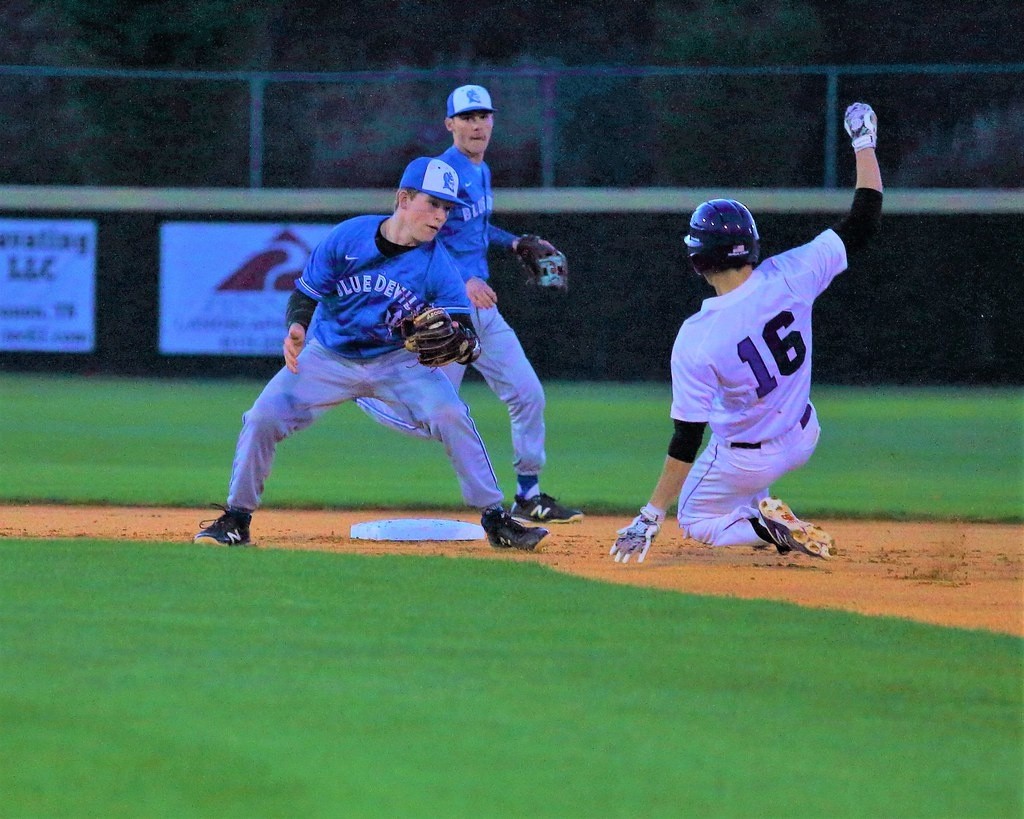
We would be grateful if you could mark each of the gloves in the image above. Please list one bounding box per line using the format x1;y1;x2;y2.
845;103;878;153
610;503;666;564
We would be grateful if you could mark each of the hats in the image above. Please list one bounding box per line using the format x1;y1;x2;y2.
447;84;498;117
399;157;473;210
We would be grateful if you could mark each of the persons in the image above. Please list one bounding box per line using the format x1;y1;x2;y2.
606;102;885;565
193;156;551;552
351;83;587;525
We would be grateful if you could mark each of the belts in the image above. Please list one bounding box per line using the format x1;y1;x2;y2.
732;403;812;449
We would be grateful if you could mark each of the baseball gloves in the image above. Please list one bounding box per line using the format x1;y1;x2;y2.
398;306;478;375
516;232;570;309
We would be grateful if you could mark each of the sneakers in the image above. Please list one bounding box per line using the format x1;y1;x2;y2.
759;496;837;562
510;493;585;523
482;507;551;552
194;502;252;545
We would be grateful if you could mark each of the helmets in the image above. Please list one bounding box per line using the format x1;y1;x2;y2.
684;198;760;275
